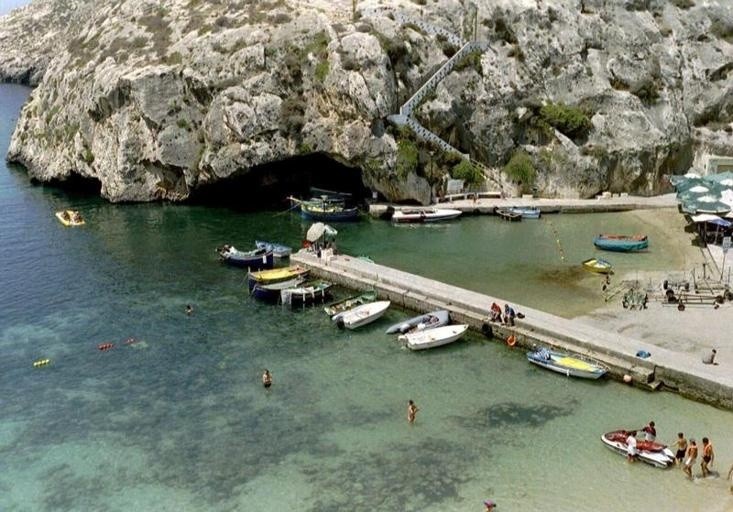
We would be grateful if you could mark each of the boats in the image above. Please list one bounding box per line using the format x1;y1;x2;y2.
300;190;360;221
248;264;333;304
385;309;469;350
323;288;391;330
582;258;614;275
55;209;85;227
593;233;648;253
392;206;462;223
526;348;608;379
601;430;675;468
495;207;540;222
215;240;293;267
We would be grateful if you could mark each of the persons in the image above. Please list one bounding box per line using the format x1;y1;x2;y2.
681;439;698;478
263;369;271;388
185;305;193;313
503;304;516;325
491;302;502;322
626;431;638;462
407;400;418;423
640;421;657;443
700;437;715;477
670;432;688;464
727;465;733;493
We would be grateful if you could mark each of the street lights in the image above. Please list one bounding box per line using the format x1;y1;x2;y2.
427;150;435;205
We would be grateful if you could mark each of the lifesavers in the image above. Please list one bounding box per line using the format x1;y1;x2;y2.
507;336;516;346
